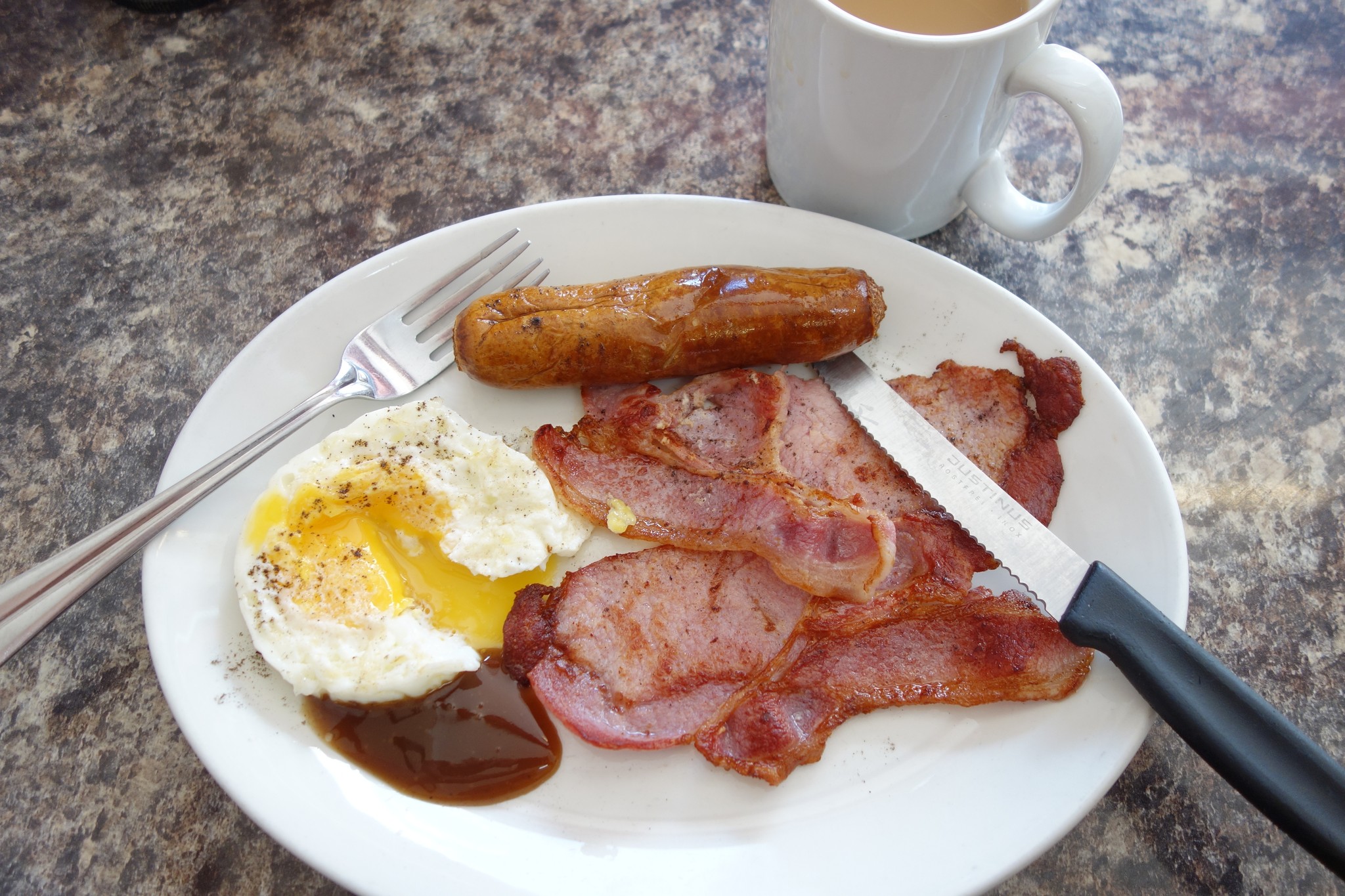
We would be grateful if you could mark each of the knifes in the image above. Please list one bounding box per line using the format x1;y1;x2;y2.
804;353;1345;882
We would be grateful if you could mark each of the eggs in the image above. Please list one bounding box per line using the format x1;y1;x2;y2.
232;395;593;704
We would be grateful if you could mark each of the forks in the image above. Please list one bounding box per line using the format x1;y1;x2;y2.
1;222;554;667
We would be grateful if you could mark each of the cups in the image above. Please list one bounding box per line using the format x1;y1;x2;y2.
764;0;1124;241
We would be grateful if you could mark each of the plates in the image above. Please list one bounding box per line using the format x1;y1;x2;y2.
140;192;1193;896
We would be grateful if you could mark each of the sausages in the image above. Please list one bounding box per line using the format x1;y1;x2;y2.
453;267;888;388
